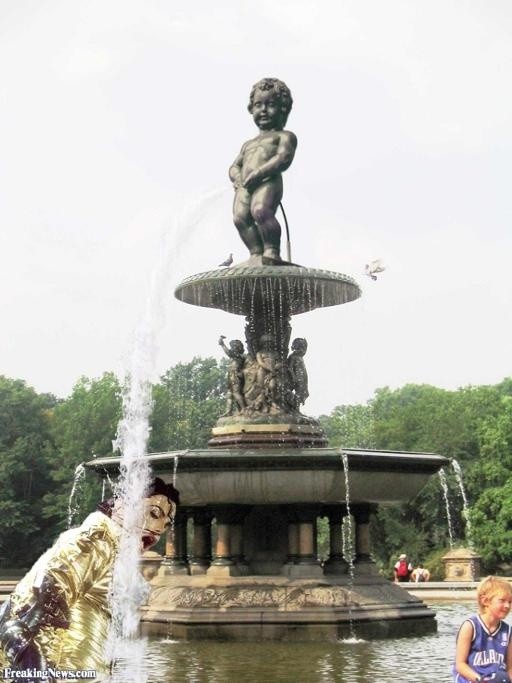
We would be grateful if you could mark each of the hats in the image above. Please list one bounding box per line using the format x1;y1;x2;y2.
399;554;407;559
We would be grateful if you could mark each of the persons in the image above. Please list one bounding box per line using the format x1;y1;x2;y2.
452;575;512;683
230;79;298;265
256;333;284;413
287;338;309;410
0;478;182;683
393;553;413;582
217;336;249;416
410;564;431;583
